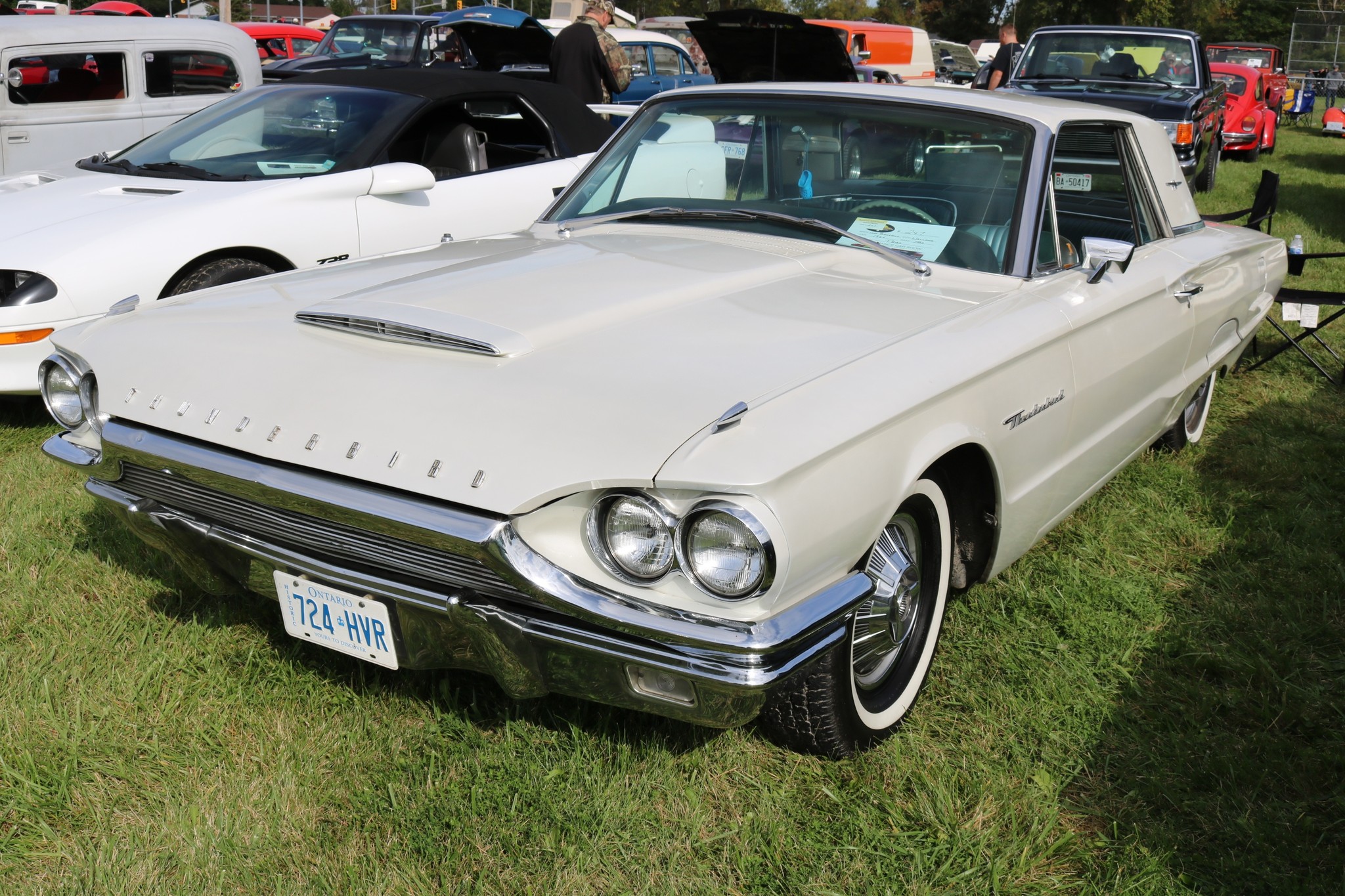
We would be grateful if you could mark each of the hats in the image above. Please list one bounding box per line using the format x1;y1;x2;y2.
587;0;618;27
1325;66;1328;69
1182;52;1191;60
278;15;281;18
281;18;286;22
1309;68;1313;71
272;19;278;23
293;18;298;22
1168;53;1176;62
1320;66;1324;69
1165;50;1173;58
1333;65;1341;69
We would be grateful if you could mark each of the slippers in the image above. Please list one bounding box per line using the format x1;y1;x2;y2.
798;170;813;200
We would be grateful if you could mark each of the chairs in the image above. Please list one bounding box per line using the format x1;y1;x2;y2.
956;224;1079;272
1199;170;1345;385
780;193;957;226
1005;209;1150;245
421;120;488;177
51;66;125;102
333;121;389;166
1282;89;1315;128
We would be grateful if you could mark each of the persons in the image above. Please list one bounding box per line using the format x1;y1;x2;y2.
549;0;632;121
1155;50;1191;78
985;23;1023;91
1305;65;1343;110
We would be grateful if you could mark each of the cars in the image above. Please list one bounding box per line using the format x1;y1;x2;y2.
1;3;1294;393
36;82;1288;762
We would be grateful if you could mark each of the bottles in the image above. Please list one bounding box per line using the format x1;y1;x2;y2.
1291;236;1304;253
